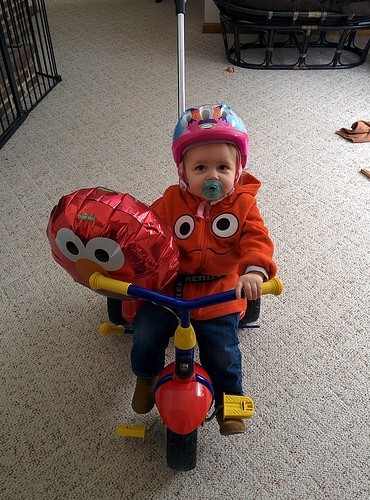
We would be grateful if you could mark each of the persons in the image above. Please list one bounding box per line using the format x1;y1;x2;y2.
121;103;278;436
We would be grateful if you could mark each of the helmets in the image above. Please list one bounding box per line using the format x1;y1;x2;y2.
170;101;251;170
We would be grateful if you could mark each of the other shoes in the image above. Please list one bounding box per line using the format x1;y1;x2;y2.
131;376;154;414
215;417;247;435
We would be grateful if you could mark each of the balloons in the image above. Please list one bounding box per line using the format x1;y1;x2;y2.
45;186;181;297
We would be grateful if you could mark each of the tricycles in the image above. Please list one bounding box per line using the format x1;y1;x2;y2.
89;0;283;473
